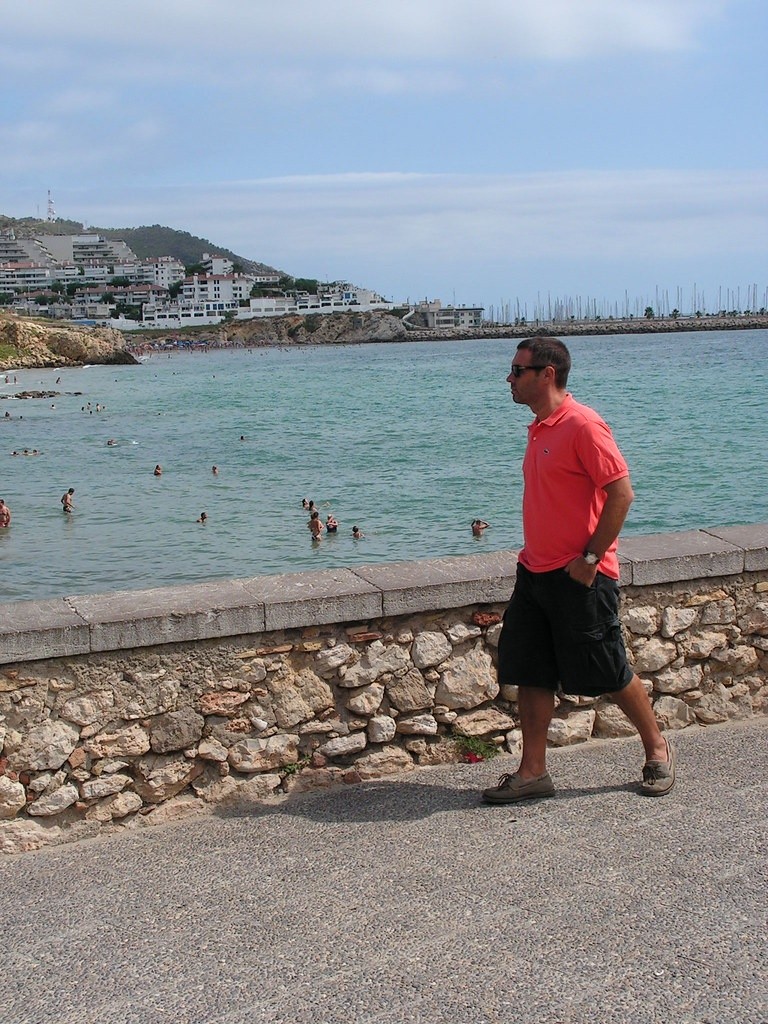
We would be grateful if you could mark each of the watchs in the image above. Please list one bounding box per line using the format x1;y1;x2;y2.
583;551;600;565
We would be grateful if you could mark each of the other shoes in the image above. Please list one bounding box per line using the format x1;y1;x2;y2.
641;738;675;796
482;772;555;803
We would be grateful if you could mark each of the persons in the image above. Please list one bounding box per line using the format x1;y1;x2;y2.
307;511;324;542
470;518;490;536
479;336;677;806
301;498;309;509
351;526;364;539
212;466;218;476
153;464;162;476
196;512;209;523
306;500;316;512
0;500;11;528
325;514;338;533
60;488;75;513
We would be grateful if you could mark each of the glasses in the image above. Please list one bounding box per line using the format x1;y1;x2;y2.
512;365;545;376
